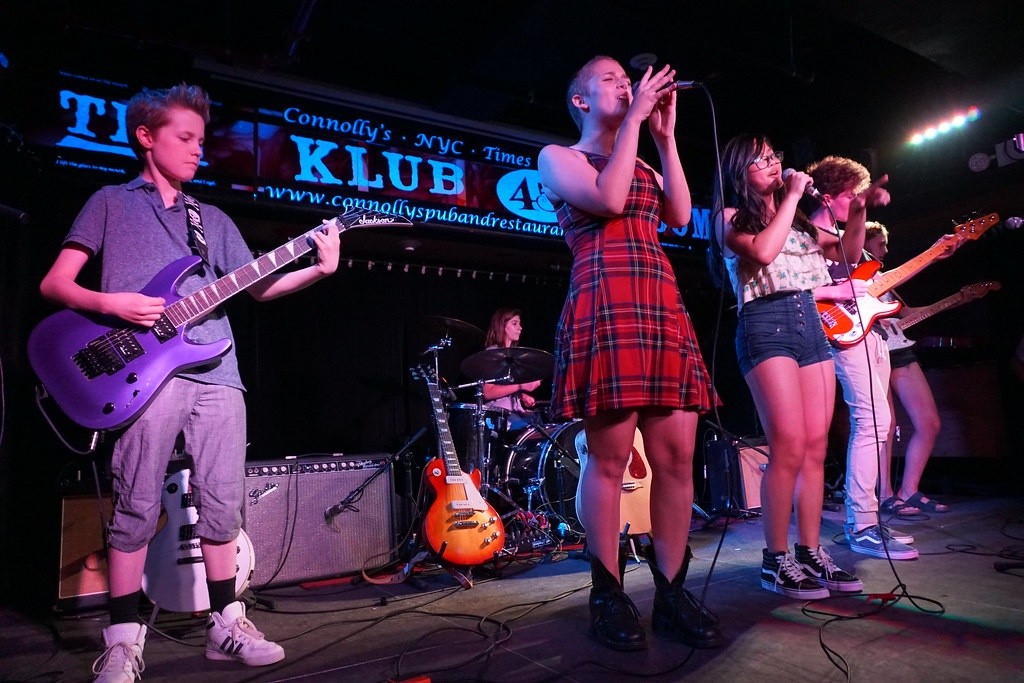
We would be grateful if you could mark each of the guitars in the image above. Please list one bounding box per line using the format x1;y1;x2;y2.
575;425;652;535
409;362;505;567
815;208;1000;350
26;207;414;435
879;280;1001;351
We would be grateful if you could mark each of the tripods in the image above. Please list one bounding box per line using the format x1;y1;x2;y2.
340;424;428;586
449;361;587;563
702;419;773;529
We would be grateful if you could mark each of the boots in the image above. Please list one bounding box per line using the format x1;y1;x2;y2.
644;541;720;646
583;541;645;650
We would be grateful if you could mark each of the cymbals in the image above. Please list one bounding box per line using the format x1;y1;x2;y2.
415;315;486;342
461;347;555;382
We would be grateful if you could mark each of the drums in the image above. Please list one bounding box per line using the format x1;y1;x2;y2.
506;422;586;538
446;402;510;490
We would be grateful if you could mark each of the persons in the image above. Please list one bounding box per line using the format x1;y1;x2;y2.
709;132;889;600
802;157;969;560
538;56;724;647
863;223;990;513
482;308;540;410
39;85;340;683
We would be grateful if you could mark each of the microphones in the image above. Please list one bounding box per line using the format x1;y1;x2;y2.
782;168;827;202
632;80;703;94
441;378;457;403
325;503;349;518
758;463;768;472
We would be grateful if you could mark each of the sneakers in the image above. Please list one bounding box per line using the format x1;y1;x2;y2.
90;623;148;683
763;547;834;600
205;601;285;666
849;526;919;561
843;517;914;546
796;541;863;591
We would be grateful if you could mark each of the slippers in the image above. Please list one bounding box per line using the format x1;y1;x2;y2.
900;492;947;513
879;494;923;516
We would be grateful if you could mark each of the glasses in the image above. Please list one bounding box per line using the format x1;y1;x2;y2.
749;152;784;169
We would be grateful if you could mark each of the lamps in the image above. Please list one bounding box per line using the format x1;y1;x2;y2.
969;134;1024;171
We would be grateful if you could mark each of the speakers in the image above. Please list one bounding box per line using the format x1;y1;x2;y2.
706;434;770;518
240;451;399;590
53;480;115;612
